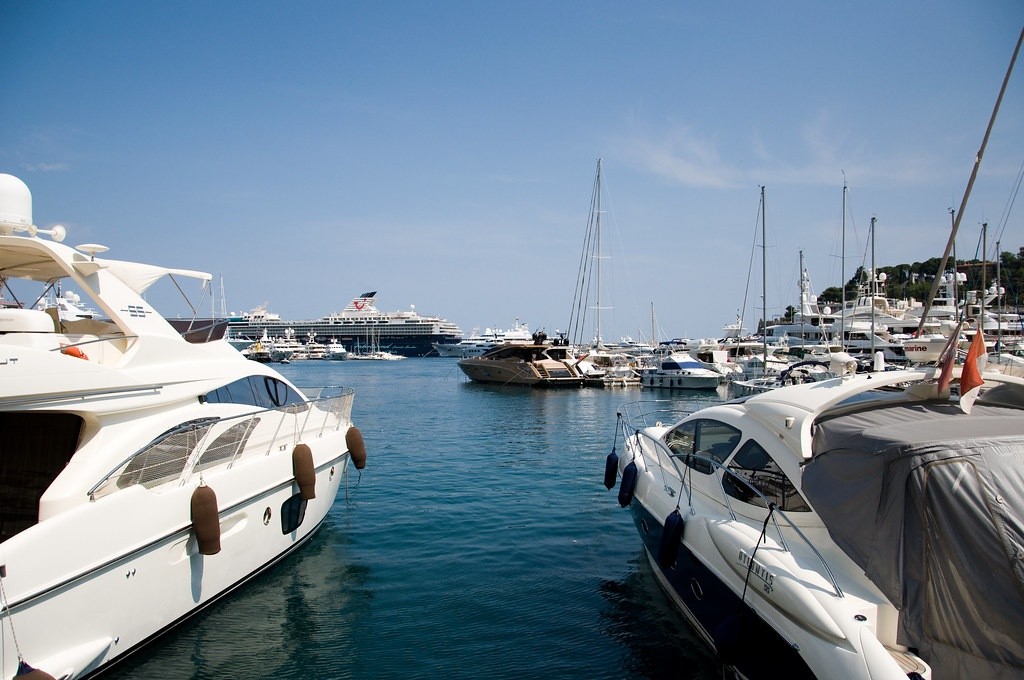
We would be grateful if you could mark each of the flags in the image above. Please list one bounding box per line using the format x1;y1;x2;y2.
960;326;988;415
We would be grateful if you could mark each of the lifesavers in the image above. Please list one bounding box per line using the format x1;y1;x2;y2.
63;346;88;360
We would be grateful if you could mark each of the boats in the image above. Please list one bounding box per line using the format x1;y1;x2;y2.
604;353;1024;680
0;175;367;680
240;325;408;363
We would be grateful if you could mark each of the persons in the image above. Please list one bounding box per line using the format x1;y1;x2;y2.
534;332;543;345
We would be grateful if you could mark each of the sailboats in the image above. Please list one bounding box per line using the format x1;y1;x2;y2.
454;26;1024;393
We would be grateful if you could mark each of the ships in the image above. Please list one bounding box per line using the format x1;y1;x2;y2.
223;292;461;356
